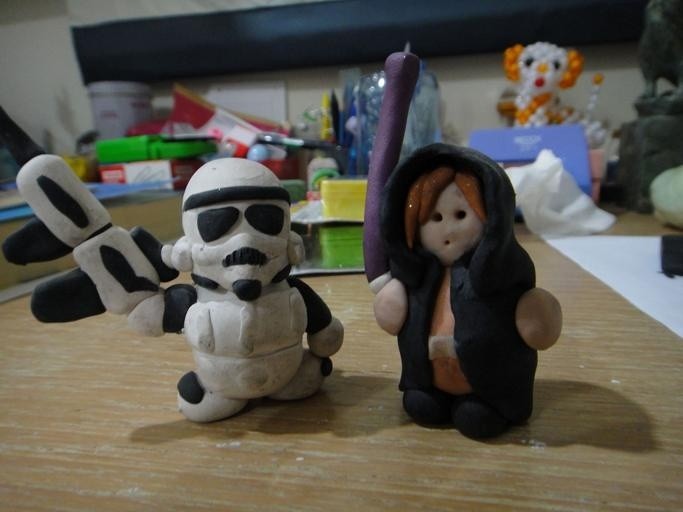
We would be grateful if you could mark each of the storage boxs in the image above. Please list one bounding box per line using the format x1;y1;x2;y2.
320;179;367;219
98;157;202;191
317;225;366;269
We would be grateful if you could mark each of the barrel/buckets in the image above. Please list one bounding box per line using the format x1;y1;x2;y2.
86;80;152;137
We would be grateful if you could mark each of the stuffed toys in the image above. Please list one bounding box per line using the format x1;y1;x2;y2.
2;149;343;424
362;45;562;441
